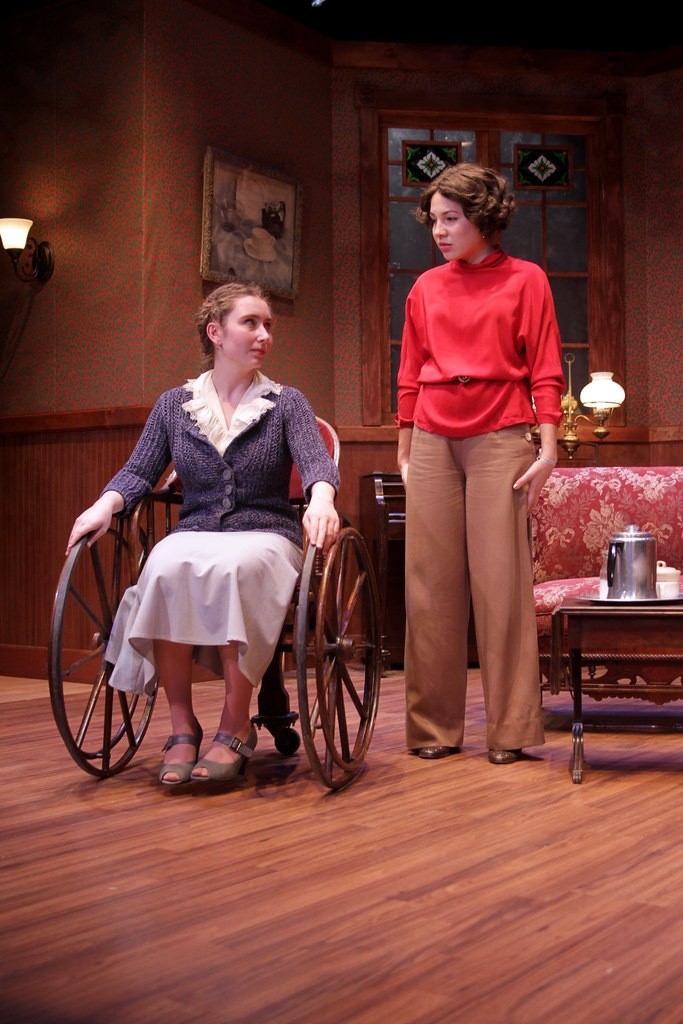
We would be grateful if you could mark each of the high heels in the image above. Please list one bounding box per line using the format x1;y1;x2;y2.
190;719;259;783
158;715;205;784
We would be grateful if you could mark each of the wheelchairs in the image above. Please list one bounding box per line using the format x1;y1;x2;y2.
47;415;382;788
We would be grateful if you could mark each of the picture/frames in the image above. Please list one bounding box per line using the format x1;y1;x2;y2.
199;145;305;301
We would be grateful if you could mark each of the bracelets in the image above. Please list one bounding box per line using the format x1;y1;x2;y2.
537;451;558;466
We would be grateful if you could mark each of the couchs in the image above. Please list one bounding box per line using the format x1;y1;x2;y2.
529;466;683;682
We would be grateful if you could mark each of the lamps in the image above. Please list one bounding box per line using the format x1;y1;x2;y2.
523;353;626;464
0;218;55;284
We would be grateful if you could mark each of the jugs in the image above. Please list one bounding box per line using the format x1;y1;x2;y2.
607;525;657;600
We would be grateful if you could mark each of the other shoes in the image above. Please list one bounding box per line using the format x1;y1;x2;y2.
418;746;458;758
487;749;521;765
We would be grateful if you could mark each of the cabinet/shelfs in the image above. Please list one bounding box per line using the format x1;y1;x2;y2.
357;472;479;669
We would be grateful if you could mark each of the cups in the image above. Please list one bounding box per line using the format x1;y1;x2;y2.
656;582;678;598
657;560;681;582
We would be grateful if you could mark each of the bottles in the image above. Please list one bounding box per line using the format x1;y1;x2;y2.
600;551;607;600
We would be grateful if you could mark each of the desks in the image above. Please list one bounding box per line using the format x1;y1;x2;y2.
551;597;683;786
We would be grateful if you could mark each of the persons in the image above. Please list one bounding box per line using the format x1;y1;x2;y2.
394;161;562;765
64;281;341;789
